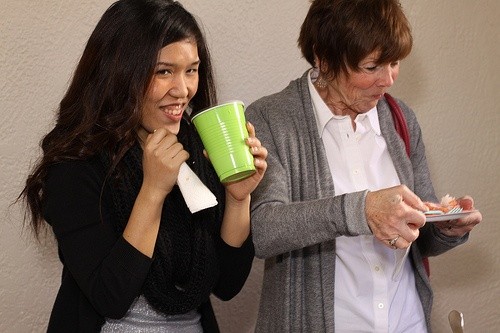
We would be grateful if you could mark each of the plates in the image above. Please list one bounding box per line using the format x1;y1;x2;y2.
425;211;473;222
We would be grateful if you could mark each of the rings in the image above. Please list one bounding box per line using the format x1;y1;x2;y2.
388;236;398;246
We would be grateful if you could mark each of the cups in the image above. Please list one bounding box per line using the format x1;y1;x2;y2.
191;100;257;183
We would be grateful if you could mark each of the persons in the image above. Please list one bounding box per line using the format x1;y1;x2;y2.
10;0;268;333
242;0;481;333
